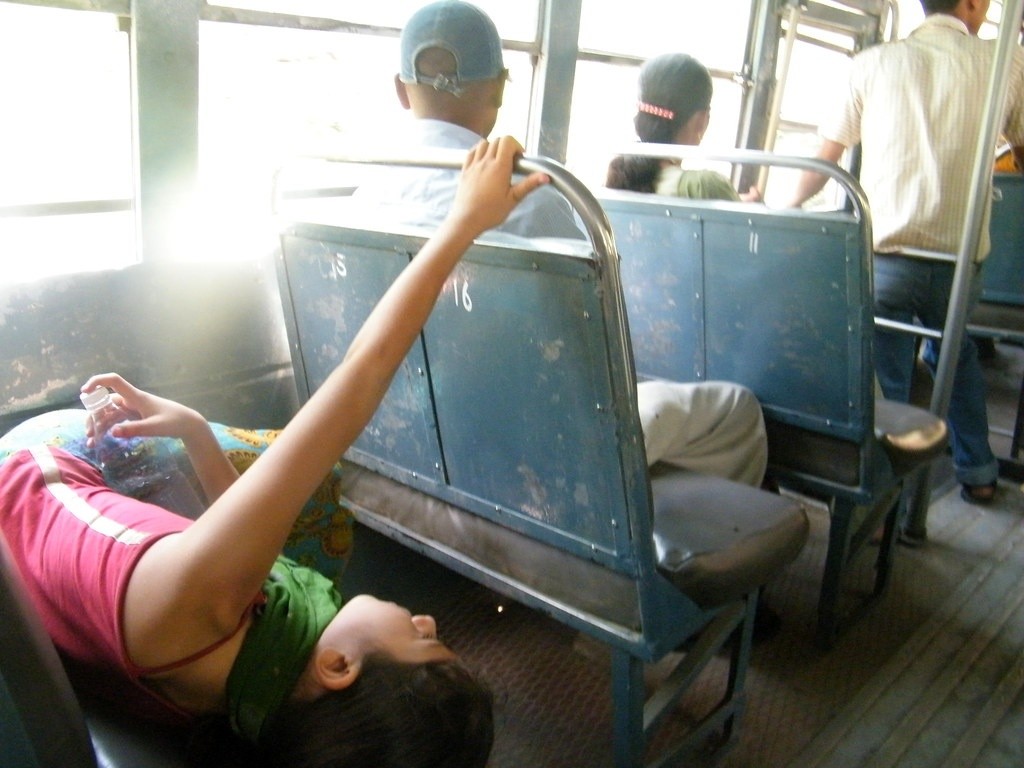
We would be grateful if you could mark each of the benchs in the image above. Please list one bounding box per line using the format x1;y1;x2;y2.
0;143;1024;768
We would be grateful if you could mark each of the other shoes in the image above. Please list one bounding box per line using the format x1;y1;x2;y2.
959;480;996;502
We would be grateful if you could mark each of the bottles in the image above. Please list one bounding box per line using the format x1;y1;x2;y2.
79;384;206;521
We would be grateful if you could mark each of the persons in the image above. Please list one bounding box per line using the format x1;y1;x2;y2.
603;50;745;205
1;134;553;768
740;0;1024;506
273;0;782;643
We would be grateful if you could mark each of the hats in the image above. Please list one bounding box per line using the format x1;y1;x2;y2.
398;0;512;97
